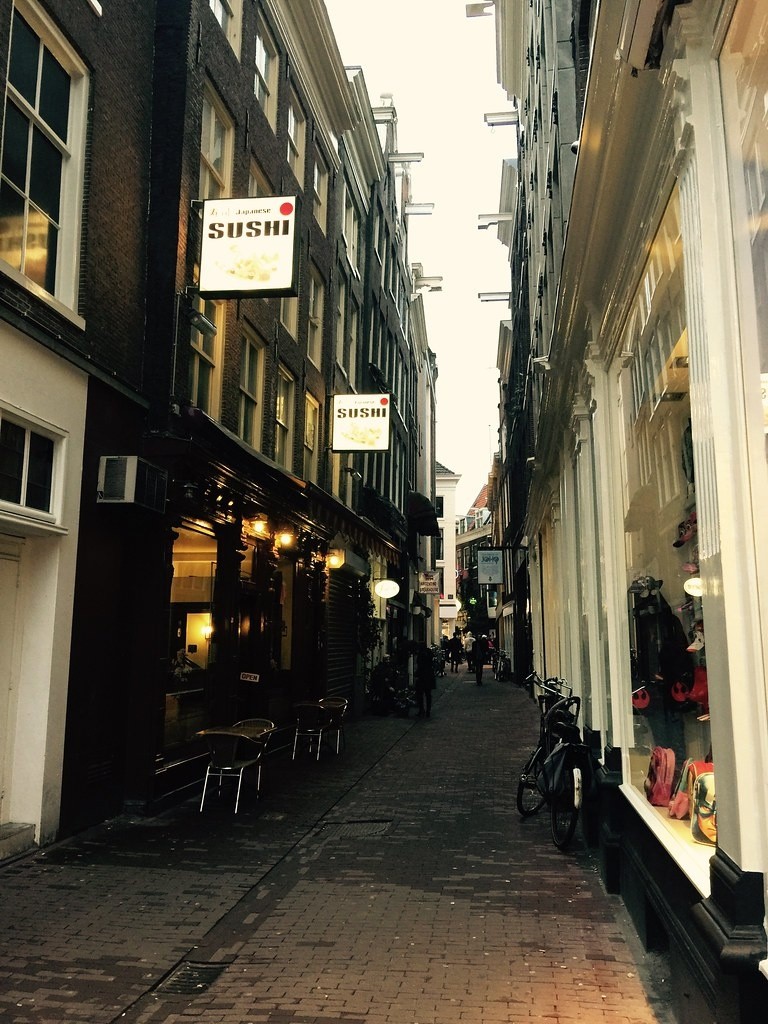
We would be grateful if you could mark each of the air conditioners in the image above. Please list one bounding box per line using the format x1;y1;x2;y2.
96;455;171;518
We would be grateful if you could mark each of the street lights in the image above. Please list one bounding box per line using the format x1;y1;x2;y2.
459;627;463;643
466;613;471;626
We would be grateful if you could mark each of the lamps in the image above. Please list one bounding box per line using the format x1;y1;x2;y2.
683;576;703;599
373;576;400;598
270;529;294;548
326;553;342;570
200;624;214;642
346;467;362;482
247;513;268;534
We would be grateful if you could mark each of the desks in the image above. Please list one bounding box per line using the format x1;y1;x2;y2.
301;701;345;752
197;727;274;805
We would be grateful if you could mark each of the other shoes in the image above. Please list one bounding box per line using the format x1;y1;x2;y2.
414;710;429;720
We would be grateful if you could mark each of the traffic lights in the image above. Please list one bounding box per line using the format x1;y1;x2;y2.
470;597;477;605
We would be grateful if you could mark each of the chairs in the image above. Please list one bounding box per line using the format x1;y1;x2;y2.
198;729;262;810
304;697;348;755
215;719;275;801
289;703;332;758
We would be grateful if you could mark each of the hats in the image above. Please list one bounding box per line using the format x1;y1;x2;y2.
673;520;697;548
685;620;704;652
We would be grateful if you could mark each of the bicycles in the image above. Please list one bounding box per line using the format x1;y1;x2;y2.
493;651;506;681
516;671;592;849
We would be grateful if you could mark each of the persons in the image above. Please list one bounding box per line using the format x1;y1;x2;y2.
413;642;437;717
440;631;493;686
633;577;694;768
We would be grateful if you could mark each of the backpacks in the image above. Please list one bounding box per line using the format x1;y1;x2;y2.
644;745;675;807
669;758;717;846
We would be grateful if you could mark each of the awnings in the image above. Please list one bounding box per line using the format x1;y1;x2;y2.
192;410;402;568
410;490;439;536
